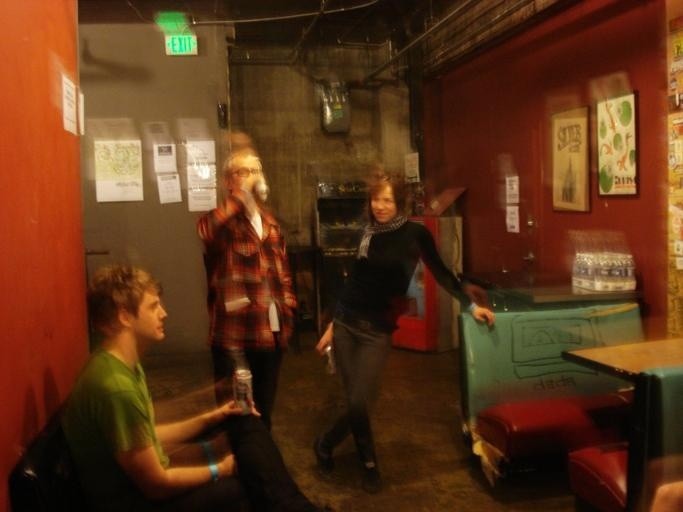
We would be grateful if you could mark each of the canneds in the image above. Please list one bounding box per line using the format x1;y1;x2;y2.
325;344;337;375
255;177;271;201
232;367;253;417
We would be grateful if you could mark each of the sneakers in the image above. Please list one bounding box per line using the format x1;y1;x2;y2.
358;454;384;487
313;438;333;481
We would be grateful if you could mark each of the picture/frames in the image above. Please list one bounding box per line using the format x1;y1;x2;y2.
550;104;590;213
595;91;639;195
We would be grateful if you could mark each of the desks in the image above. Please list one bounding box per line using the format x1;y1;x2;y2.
561;338;683;512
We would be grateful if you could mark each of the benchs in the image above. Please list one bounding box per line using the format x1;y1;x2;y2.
460;301;646;502
569;367;683;512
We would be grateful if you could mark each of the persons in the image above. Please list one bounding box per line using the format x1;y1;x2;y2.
198;147;300;434
63;262;333;512
310;164;499;498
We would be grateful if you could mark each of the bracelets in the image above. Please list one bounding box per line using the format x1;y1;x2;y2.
235;185;250;197
464;302;478;314
207;461;218;482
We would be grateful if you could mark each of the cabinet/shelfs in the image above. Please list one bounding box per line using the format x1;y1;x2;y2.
314;179;371;336
